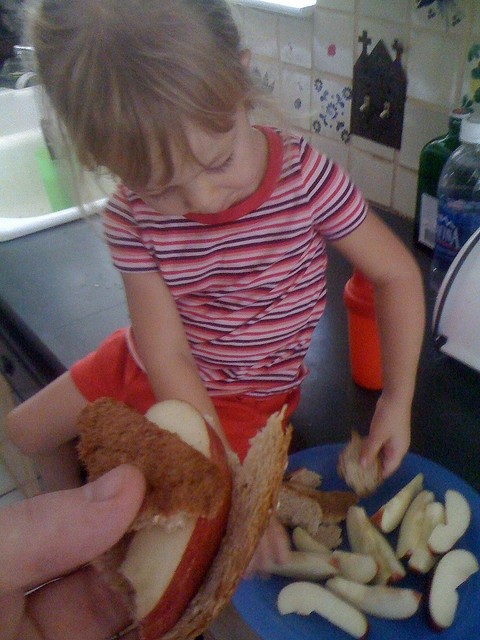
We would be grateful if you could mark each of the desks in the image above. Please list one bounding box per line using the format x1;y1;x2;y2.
0;199;480;500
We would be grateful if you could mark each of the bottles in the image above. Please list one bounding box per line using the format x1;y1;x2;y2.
414;116;464;255
428;117;479;317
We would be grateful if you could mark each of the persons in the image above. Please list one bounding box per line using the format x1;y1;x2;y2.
0;463;215;640
4;1;426;482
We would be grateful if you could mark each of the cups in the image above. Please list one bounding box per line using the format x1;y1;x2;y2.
35;146;80;213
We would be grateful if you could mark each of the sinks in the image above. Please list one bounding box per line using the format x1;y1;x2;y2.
0;132;122;243
0;85;41;138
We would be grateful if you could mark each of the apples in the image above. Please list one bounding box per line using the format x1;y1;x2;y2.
334;551;378;582
327;577;422;621
293;528;339;568
266;553;337;581
409;502;445;576
424;549;480;631
279;582;369;639
346;505;406;585
394;489;435;559
427;489;471;555
370;472;425;533
119;399;232;639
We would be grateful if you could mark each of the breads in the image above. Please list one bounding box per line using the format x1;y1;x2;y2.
280;469;356;550
76;398;223;532
157;406;293;639
338;432;382;497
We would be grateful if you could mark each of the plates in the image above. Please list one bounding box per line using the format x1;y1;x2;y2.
224;442;480;640
